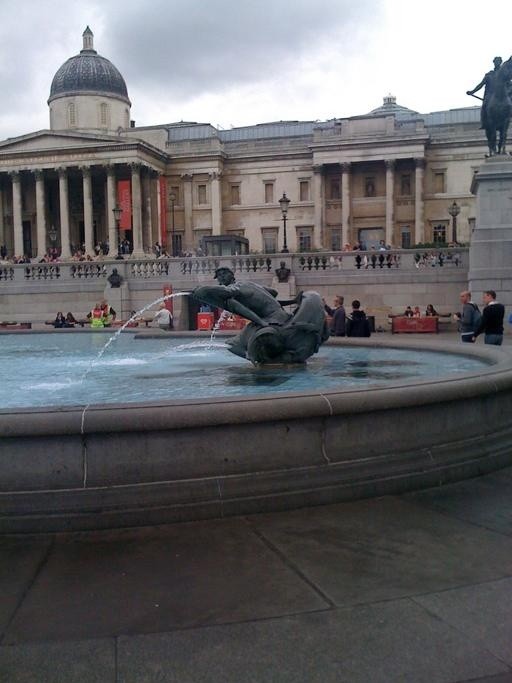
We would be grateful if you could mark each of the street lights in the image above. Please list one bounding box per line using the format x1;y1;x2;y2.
112;204;124;260
449;201;461;244
47;225;58;259
277;190;291;252
169;191;177;259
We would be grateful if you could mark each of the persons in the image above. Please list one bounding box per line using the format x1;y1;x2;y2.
86;302;108;328
339;239;462;269
125;310;140;327
1;238;205;280
344;300;373;338
64;311;79;328
425;303;440;316
53;311;66;328
466;56;503;157
274;261;291;283
472;290;506;346
192;265;304;328
453;290;482;343
320;294;347;337
100;299;117;327
107;268;123;288
154;301;173;330
412;306;423;317
404;305;414;317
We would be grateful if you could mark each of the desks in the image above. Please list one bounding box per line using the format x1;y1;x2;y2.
392;315;439;334
0;318;153;329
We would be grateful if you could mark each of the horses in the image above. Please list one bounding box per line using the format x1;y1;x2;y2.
482;56;512;156
